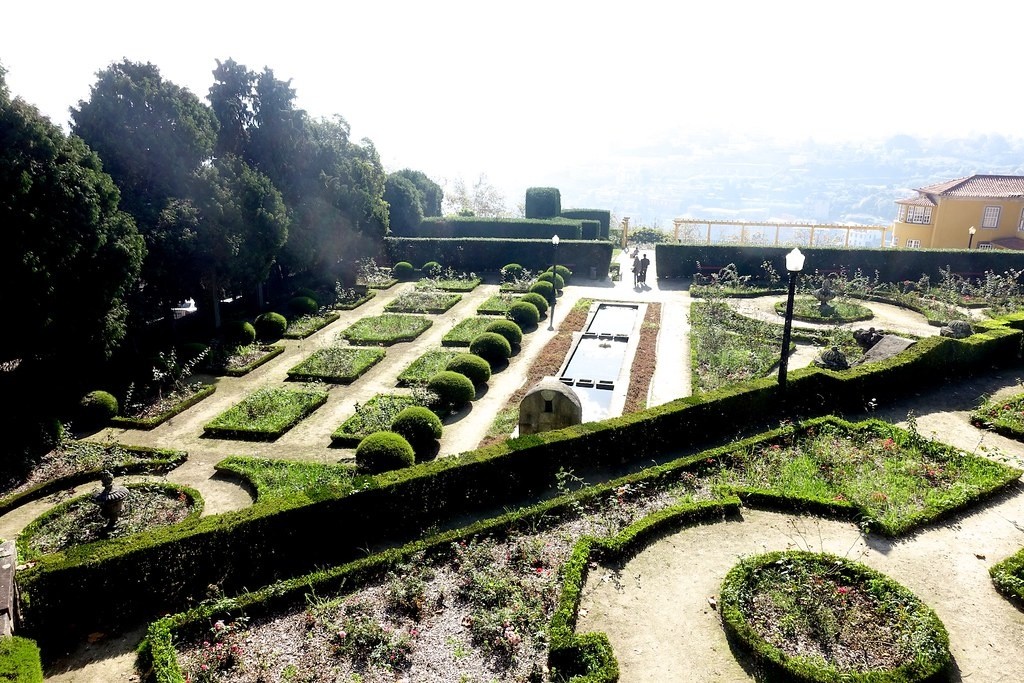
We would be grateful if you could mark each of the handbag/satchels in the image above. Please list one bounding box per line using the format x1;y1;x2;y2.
637;273;645;282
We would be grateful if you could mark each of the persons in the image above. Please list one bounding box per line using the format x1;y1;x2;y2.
632;255;640;288
639;253;650;285
633;246;639;256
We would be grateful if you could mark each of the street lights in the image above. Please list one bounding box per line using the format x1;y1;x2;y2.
778;246;807;409
967;225;976;249
551;234;560;303
